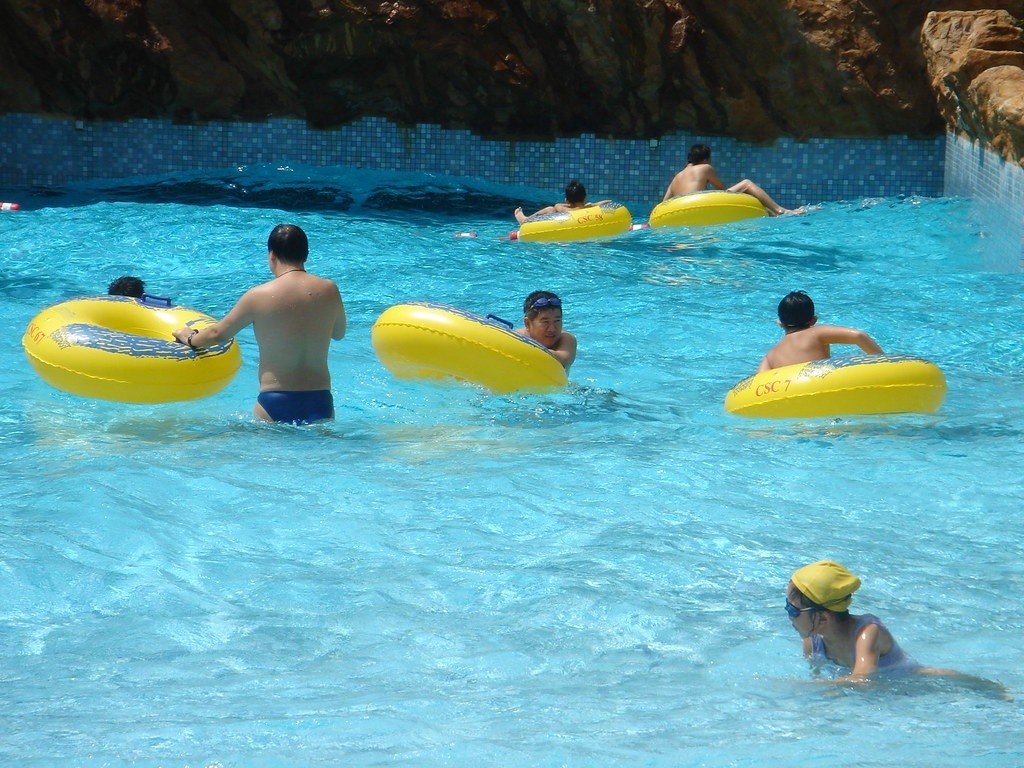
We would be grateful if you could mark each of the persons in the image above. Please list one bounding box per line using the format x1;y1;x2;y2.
663;144;805;216
514;181;612;225
785;560;1012;701
514;290;577;378
758;290;883;374
172;223;346;425
108;276;145;298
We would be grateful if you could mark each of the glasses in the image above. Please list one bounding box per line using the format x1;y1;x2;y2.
524;297;562;317
785;599;813;619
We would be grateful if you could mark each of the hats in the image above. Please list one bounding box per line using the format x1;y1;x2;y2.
790;561;860;612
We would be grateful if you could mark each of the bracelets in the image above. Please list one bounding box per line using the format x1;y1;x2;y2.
188;329;199;348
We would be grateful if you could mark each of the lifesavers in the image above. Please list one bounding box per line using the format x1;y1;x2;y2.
649;189;769;230
20;294;243;406
371;303;566;394
725;355;948;419
520;201;632;242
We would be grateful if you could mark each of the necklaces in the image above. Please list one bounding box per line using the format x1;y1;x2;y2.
277;269;306;278
